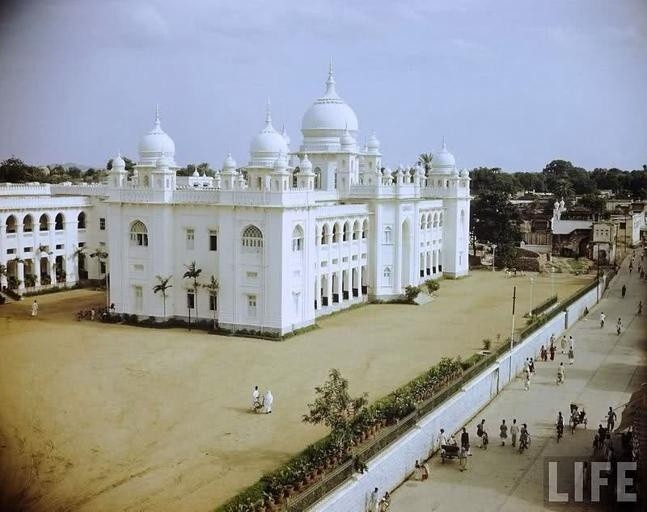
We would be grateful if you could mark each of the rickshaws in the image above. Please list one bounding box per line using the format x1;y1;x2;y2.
440;439;474;469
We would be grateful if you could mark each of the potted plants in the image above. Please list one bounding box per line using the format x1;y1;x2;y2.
223;356;463;512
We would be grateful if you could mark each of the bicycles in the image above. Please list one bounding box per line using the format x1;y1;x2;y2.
556;421;564;444
253;396;266;412
554;372;567;386
616;323;625;336
600;316;606;329
518;433;532;455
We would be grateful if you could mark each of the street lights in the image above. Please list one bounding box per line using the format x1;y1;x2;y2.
492;244;496;271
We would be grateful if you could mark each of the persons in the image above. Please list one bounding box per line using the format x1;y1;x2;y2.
477;419;486;448
264;390;272;413
460;427;469;451
557;404;617;457
509;419;519;447
89;308;96;320
370;487;379;512
414;459;420;480
517;423;529;449
498;418;508;446
383;491;390;512
250;385;260;411
31;299;38;316
438;428;447;451
519;331;576;389
599;249;647;337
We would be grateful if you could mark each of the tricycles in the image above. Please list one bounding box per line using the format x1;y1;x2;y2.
569;416;588;435
